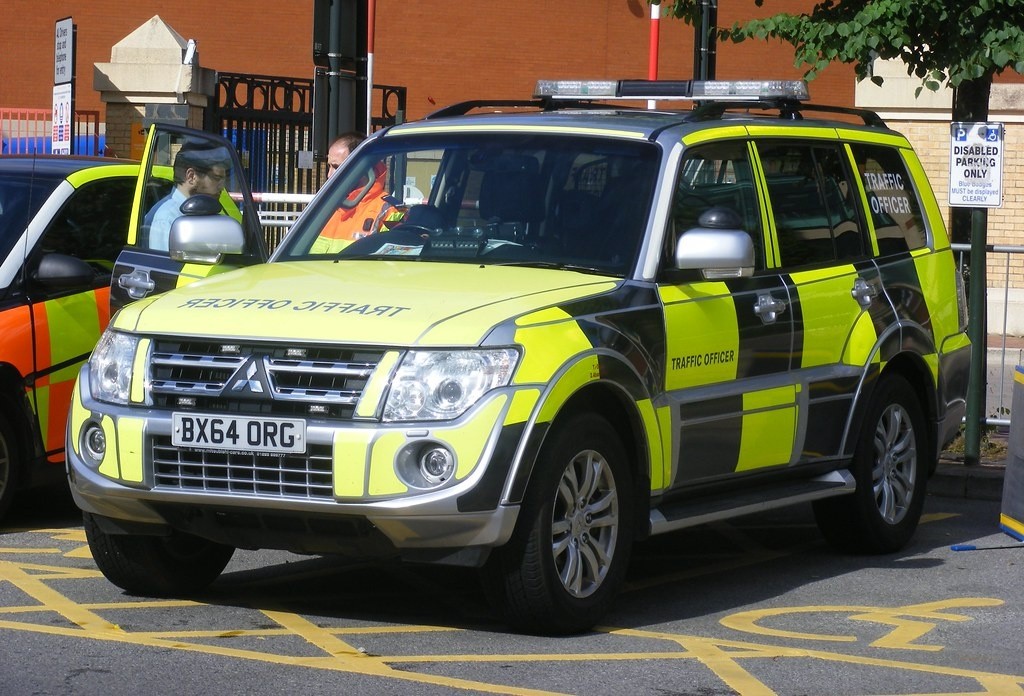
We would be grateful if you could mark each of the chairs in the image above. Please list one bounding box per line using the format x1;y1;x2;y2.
480;155;564;257
69;185;127;261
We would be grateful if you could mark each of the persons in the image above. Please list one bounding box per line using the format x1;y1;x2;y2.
141;136;231;251
308;131;410;254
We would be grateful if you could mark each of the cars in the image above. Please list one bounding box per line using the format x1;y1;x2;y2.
0;153;248;526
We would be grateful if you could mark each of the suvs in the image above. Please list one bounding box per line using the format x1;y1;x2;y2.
64;80;974;638
